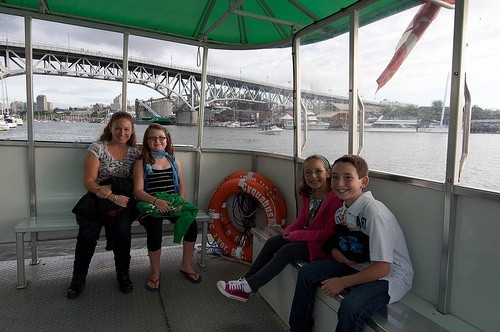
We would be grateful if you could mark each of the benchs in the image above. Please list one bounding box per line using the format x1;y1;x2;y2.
14;207;212;291
248;224;451;332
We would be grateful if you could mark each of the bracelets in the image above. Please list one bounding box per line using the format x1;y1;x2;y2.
153;199;157;204
112;195;118;203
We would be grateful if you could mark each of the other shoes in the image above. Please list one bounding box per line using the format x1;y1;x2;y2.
66;281;85;297
117;272;133;293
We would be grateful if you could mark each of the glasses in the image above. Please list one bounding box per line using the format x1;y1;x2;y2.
302;168;326;177
147;135;167;141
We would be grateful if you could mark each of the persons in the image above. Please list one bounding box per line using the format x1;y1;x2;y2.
133;124;202;291
67;110;141;299
216;154;343;303
289;155;415;332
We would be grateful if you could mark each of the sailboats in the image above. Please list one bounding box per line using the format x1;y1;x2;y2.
1;80;17;128
33;112;89;124
417;71;449;133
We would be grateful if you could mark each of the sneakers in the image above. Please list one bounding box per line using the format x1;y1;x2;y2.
216;278;252;302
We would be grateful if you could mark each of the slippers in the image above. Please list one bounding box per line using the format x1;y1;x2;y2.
146;273;161;291
180;267;202;284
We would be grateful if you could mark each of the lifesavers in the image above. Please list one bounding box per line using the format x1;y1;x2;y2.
216;168;287;248
206;177;281;261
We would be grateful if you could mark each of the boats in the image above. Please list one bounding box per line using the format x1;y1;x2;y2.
17;117;24;124
0;119;9;131
280;111;331;132
258;124;283;134
211;119;258;128
356;114;418;133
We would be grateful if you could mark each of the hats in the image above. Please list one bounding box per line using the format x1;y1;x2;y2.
333;223;370;264
